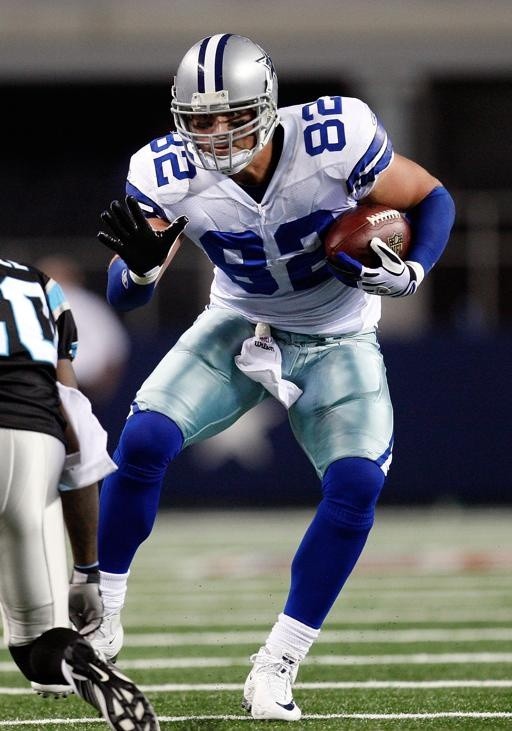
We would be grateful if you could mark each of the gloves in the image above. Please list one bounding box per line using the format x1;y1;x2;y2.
325;236;425;298
95;194;190;286
67;560;106;638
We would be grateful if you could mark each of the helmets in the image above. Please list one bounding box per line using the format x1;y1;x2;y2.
169;31;281;171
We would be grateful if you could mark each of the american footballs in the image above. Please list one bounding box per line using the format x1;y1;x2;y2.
325;203;412;273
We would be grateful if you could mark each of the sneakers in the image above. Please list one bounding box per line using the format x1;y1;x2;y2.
28;611;127;701
60;638;161;731
240;645;302;723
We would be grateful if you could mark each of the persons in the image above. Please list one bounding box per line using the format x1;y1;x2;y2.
30;32;456;721
0;257;161;731
23;226;132;402
